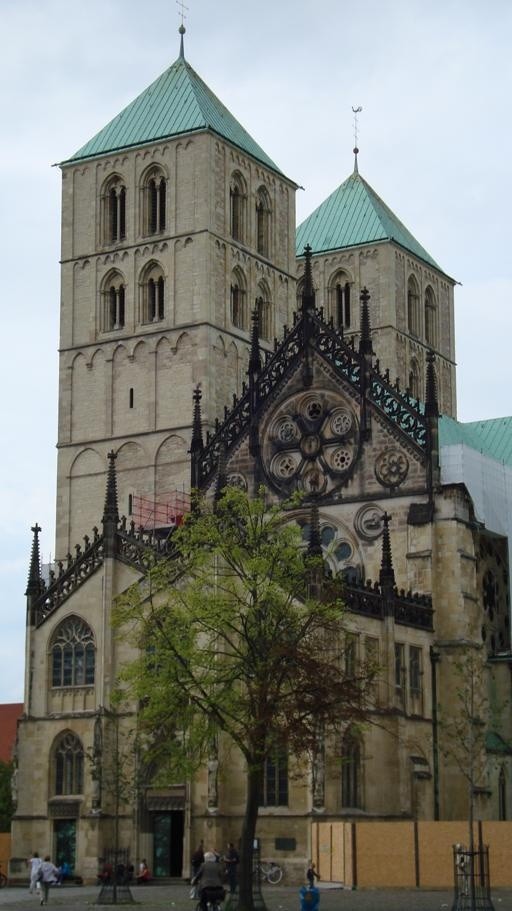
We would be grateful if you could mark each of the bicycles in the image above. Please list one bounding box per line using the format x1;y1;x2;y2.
196;901;222;911
0;865;8;889
255;861;282;885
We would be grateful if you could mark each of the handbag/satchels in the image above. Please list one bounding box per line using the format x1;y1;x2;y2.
36;869;43;882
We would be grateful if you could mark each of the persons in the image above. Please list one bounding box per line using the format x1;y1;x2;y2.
307;862;320;888
137;858;150;882
29;852;60;906
190;839;238;911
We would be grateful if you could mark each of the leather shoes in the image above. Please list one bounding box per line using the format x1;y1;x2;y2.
39;898;47;906
